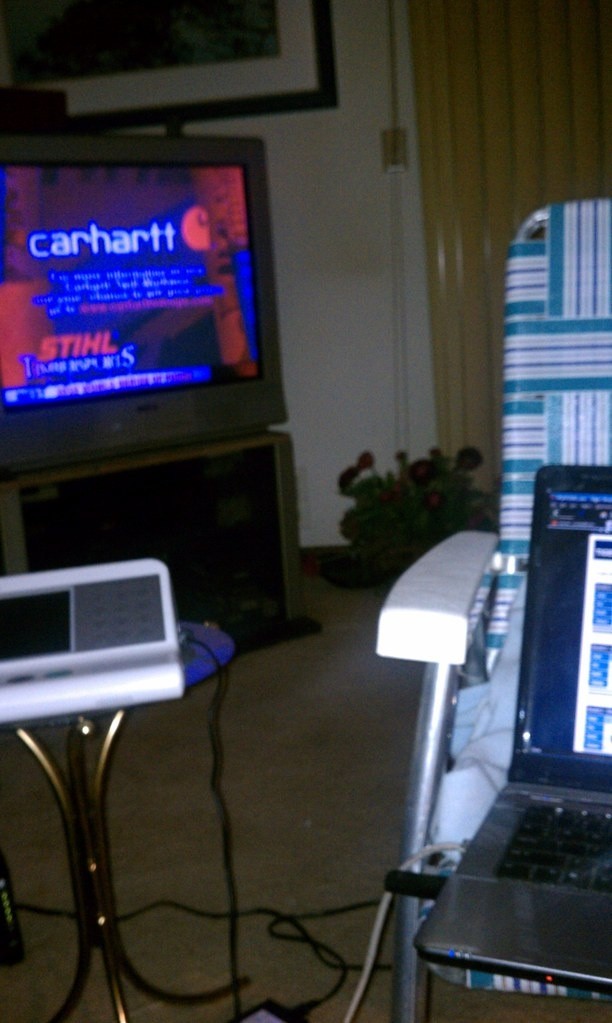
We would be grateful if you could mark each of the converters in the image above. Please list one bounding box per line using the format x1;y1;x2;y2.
229;999;309;1023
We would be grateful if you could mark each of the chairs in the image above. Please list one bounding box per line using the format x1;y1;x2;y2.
375;200;612;1023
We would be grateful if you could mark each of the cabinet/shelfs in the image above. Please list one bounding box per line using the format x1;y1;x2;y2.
0;431;324;661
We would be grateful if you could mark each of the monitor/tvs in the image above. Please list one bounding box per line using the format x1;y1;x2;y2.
0;134;289;478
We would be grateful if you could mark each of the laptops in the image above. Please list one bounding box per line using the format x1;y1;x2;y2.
413;466;612;994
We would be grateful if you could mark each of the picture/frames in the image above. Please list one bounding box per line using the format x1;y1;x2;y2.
0;0;339;132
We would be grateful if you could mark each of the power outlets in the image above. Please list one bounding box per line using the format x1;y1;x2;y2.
381;128;409;174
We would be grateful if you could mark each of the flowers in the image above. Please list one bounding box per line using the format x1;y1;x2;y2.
334;445;505;597
